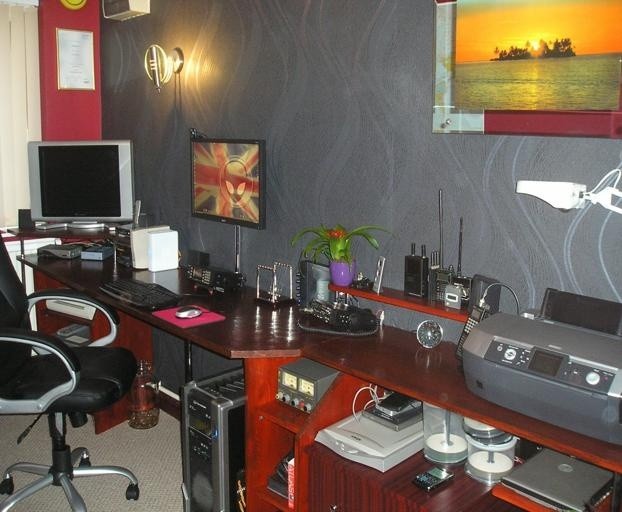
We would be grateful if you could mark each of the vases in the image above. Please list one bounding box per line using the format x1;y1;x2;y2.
329;255;355;286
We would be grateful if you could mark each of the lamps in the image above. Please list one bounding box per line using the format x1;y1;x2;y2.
143;46;186;90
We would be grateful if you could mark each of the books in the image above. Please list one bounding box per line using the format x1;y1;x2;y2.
287;458;296;509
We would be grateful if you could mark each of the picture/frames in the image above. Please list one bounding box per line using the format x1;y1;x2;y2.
56;26;96;90
433;0;622;142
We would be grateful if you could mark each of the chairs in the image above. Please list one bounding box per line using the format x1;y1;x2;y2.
0;230;142;511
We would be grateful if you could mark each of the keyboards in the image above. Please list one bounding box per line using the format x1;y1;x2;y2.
98;277;182;312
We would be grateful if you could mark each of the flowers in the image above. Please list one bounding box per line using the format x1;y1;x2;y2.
290;219;391;259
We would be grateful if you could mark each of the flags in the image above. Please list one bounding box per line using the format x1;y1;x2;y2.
193;142;259;223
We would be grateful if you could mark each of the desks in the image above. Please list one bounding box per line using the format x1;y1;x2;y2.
298;276;622;511
15;231;298;511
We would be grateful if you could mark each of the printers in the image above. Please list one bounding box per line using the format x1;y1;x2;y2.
462;286;622;449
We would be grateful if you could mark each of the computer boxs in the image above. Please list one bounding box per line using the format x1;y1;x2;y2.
181;366;245;512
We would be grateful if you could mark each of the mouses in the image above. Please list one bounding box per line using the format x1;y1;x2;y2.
175;306;202;319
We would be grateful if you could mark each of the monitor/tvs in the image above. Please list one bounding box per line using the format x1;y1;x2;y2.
27;138;134;232
